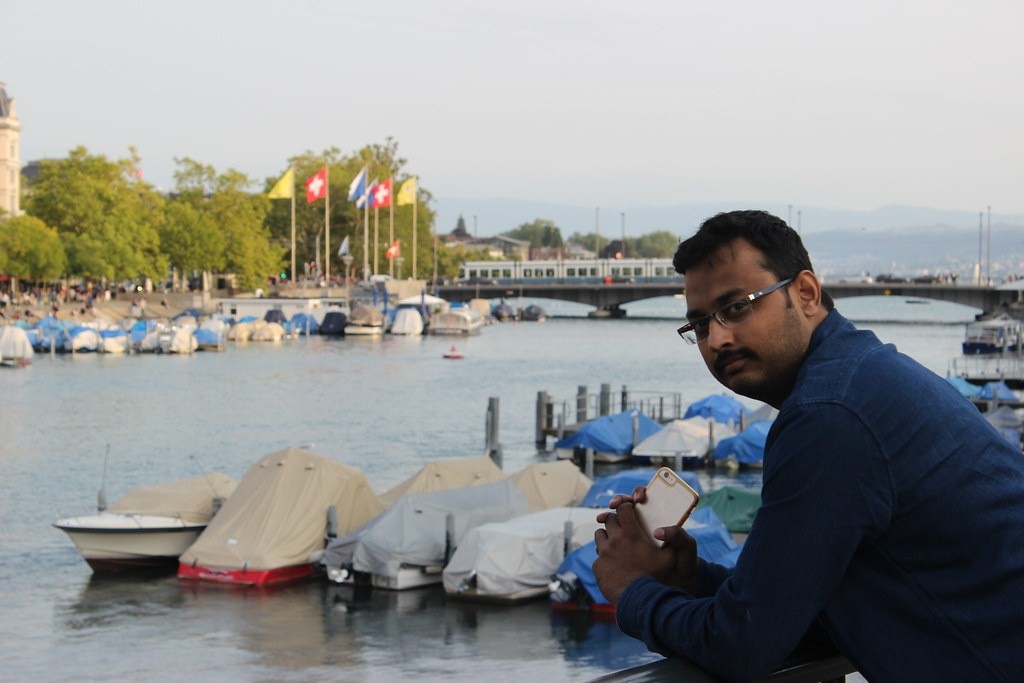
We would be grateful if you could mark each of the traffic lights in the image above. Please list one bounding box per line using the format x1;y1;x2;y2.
281;273;287;279
310;259;316;267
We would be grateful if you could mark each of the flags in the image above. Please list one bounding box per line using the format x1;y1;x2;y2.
265;166;295;199
305;165;327;204
396;175;416;206
347;166;391;208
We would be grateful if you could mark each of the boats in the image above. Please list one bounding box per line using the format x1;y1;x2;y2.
50;442;234;577
316;288;548;336
176;394;780;620
962;320;1024;355
1;316;318;366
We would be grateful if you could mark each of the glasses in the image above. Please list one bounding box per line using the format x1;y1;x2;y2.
677;278;793;347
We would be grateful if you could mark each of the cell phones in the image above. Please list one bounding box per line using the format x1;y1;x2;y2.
633;467;700;549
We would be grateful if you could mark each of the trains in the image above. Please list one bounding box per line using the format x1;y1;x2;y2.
456;259;681;281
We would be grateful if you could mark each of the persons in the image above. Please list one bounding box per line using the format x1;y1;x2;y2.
591;209;1024;683
0;284;111;326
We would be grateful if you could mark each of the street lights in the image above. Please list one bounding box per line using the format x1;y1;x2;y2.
595;207;601;278
788;204;792;224
796;208;803;238
988;204;992;276
620;211;626;259
472;215;479;263
979;213;983;286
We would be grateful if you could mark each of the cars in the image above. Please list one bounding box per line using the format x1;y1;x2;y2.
877;272;934;284
838;276;873;286
